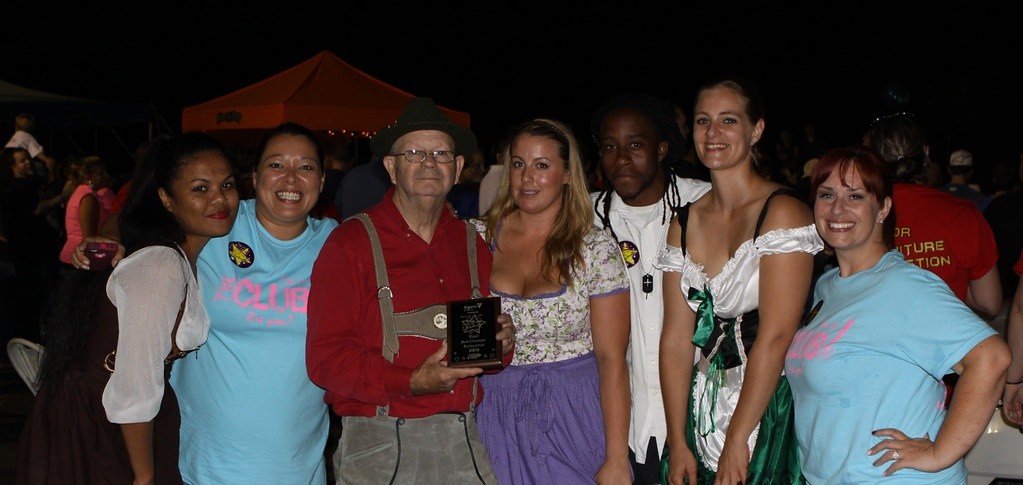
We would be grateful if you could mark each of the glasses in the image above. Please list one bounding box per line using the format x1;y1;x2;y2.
393;150;458;164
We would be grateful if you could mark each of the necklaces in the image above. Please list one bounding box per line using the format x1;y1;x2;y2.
624;184;671;293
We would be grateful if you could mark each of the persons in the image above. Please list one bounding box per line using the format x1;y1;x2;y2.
577;106;712;484
657;77;825;485
0;110;1023;427
469;120;634;485
22;132;241;485
306;97;516;484
784;145;1012;485
168;122;340;485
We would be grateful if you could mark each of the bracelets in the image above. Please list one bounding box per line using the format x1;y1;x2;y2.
1005;380;1023;385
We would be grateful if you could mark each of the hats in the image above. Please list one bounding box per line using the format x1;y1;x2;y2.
950;150;972;176
369;96;477;165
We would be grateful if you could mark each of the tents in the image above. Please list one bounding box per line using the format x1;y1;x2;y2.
182;48;470;142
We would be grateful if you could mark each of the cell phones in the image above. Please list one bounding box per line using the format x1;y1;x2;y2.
82;241;118;271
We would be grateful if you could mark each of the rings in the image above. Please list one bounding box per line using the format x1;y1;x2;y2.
891;450;900;460
